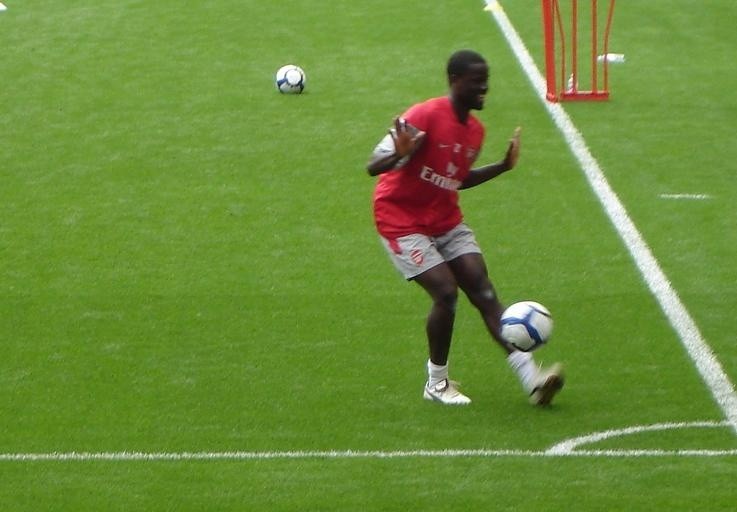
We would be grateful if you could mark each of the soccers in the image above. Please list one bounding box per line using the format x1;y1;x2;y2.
498;301;552;351
277;65;306;94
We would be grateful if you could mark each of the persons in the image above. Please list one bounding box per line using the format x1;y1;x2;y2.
366;48;566;406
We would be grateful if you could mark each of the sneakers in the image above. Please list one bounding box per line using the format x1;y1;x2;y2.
424;379;472;404
527;362;565;404
